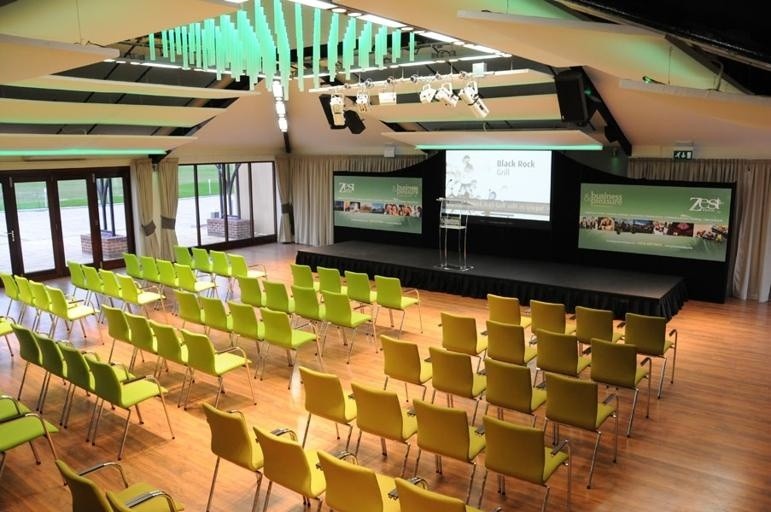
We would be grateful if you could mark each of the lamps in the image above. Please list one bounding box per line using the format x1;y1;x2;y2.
456;0;669;43
150;0;512;133
616;46;771;108
0;1;121;59
328;71;489;125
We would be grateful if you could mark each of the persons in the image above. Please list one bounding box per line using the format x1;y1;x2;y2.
581;217;589;229
384;204;422;217
590;217;597;230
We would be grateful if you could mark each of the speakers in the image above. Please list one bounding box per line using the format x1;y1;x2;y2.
554;71;589;122
344;110;366;134
319;94;348;130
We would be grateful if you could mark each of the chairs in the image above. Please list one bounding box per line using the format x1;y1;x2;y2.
55;460;181;512
1;393;69;488
203;292;677;512
0;244;425;459
